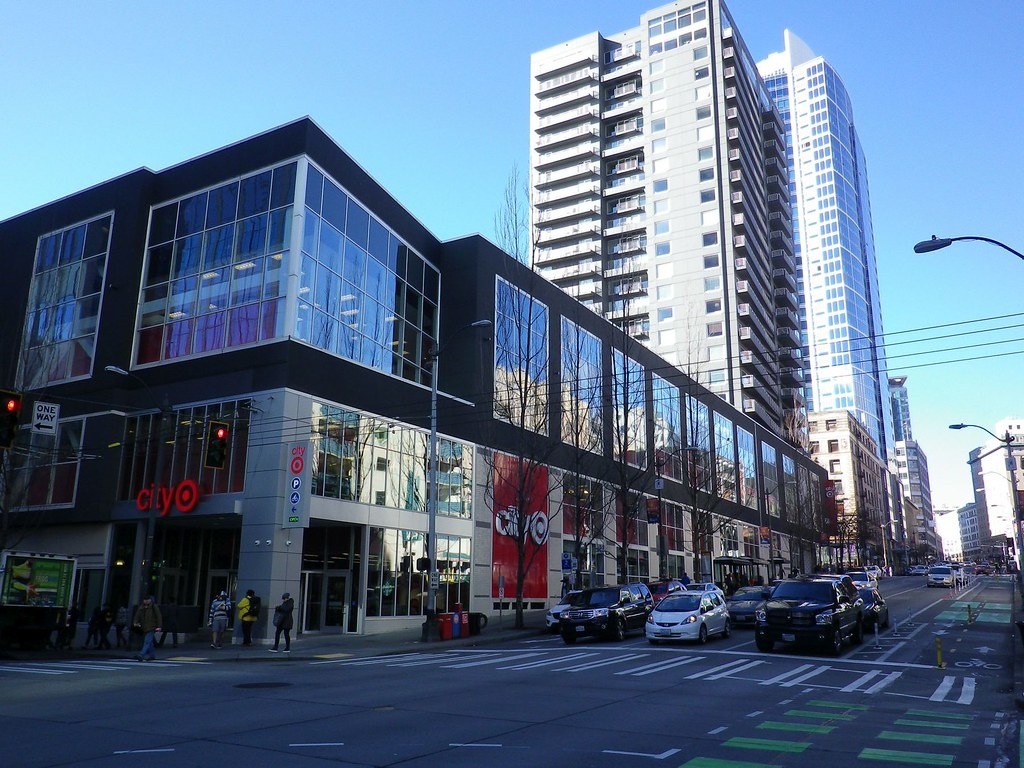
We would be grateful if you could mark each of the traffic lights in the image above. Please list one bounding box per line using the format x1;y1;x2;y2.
204;422;229;469
0;389;22;450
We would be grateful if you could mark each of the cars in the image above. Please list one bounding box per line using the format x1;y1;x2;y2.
645;581;732;646
907;561;966;588
964;559;1009;575
854;565;884;579
731;572;888;654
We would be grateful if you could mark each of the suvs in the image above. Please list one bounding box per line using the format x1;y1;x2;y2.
544;582;655;642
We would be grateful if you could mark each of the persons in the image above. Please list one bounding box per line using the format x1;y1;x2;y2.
208;591;232;650
236;588;258;647
53;593;180;662
724;564;854;596
681;573;690;586
268;593;295;653
994;560;1018;581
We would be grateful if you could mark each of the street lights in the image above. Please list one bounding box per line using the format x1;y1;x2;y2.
655;446;701;581
949;423;1023;578
429;320;494;621
880;519;899;569
764;480;798;583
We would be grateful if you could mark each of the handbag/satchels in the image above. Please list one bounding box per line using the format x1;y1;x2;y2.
271;611;284;626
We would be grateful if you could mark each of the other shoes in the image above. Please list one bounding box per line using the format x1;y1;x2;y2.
217;646;222;648
147;657;157;660
211;643;216;648
283;649;290;652
268;648;278;652
135;655;143;661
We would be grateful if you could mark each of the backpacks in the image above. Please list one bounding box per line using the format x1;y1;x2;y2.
245;596;261;616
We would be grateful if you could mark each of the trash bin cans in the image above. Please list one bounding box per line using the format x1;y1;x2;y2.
468;612;482;635
439;613;452;640
450;612;461;639
461;611;469;638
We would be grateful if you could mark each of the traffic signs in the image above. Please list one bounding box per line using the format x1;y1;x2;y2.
32;402;60;435
